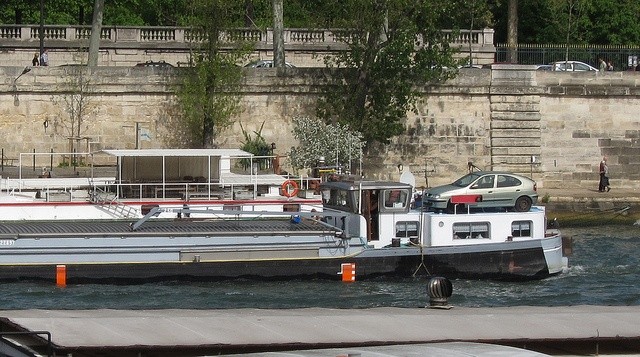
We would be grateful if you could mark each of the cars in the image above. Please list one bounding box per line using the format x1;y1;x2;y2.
422;171;538;212
244;59;296;67
536;65;565;71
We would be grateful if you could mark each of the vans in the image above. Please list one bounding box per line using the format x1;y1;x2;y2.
549;60;600;72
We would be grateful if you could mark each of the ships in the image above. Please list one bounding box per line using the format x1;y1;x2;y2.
0;148;324;218
0;126;564;282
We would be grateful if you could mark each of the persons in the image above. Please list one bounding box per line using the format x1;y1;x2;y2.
32;53;39;65
40;48;49;66
606;61;614;71
598;156;612;193
598;58;607;71
397;163;416;207
310;155;327;177
